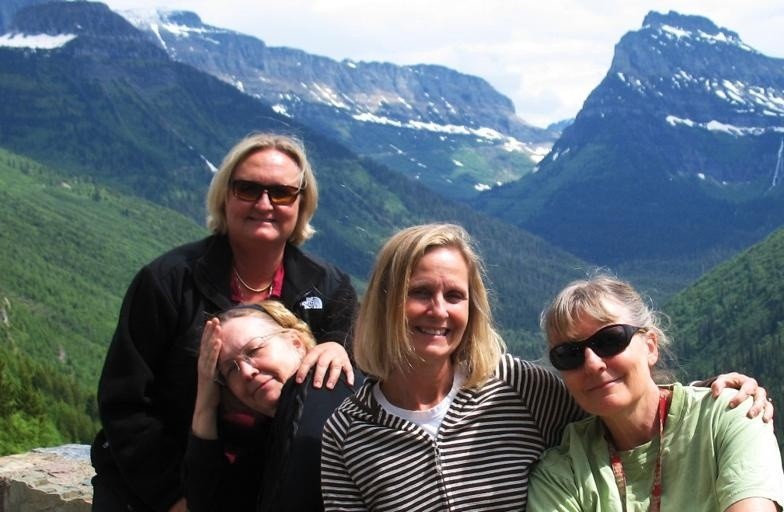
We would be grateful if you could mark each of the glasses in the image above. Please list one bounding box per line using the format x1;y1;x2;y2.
214;331;287;386
550;324;644;370
228;180;304;204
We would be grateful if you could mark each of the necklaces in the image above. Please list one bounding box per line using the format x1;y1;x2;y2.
233;266;273;293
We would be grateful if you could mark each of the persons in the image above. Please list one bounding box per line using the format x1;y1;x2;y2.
526;277;783;512
321;222;773;512
92;133;361;512
183;300;366;512
